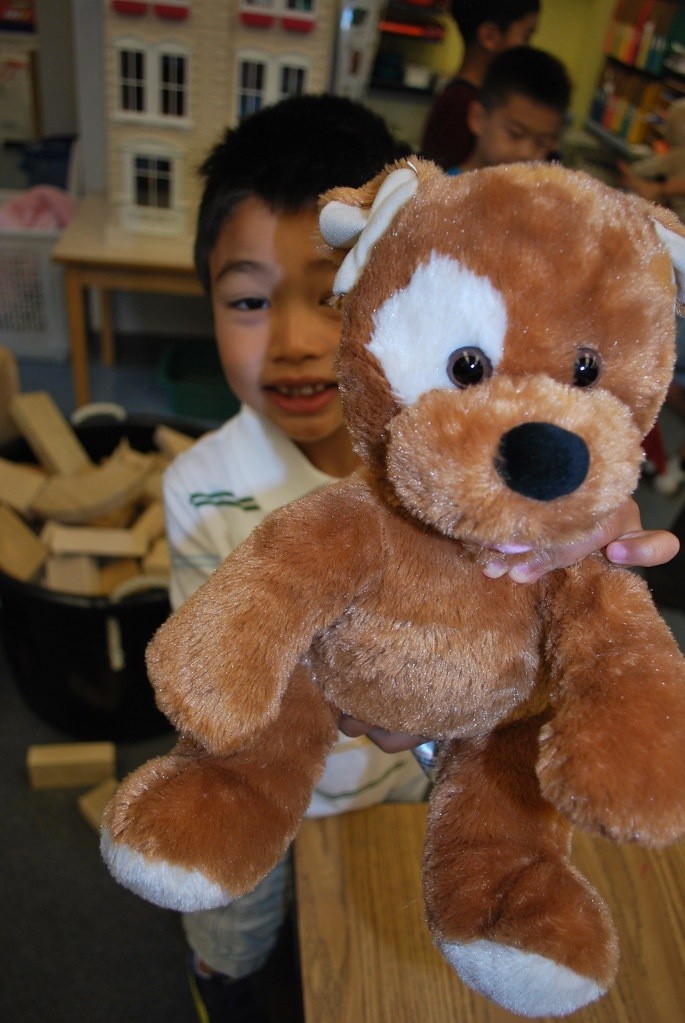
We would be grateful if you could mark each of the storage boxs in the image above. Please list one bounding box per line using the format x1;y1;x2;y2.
0;411;224;746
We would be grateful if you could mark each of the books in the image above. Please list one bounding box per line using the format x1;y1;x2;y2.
588;0;685;147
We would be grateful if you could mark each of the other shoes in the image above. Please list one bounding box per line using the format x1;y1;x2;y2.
192;950;269;1023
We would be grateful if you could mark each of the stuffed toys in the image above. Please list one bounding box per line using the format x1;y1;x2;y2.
95;155;685;1023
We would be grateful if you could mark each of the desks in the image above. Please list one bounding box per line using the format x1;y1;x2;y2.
52;199;207;407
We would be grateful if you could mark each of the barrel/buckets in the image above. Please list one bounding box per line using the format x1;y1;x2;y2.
0;404;212;743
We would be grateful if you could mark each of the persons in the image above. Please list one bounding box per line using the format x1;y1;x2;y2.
158;92;685;1015
421;0;685;497
